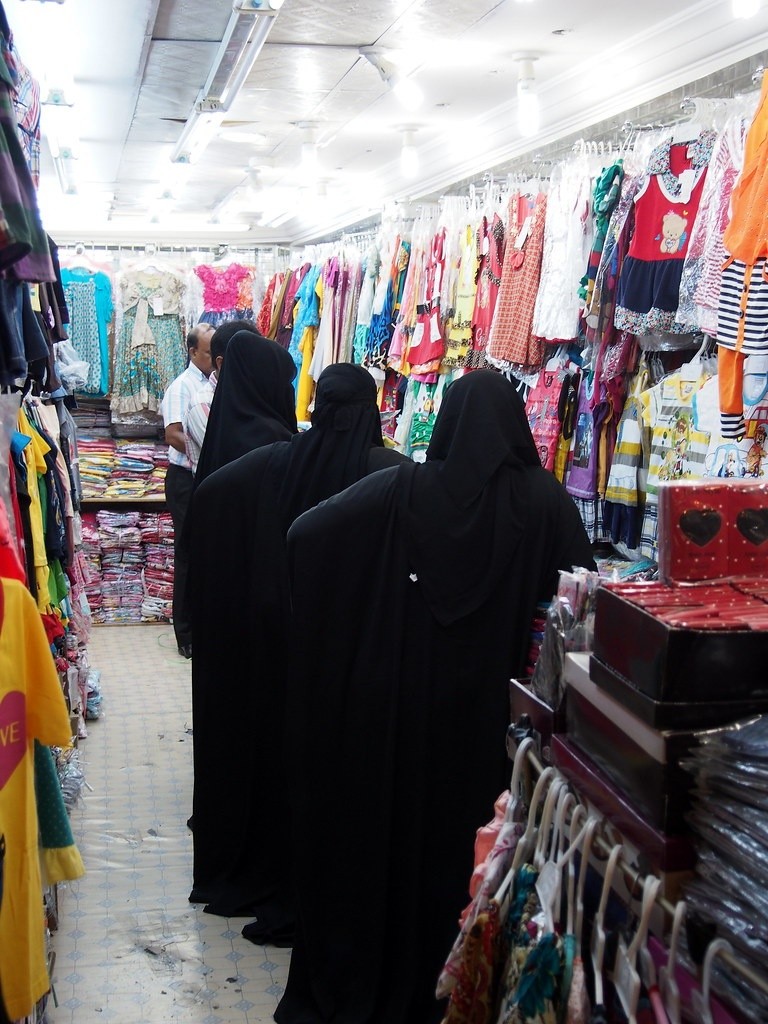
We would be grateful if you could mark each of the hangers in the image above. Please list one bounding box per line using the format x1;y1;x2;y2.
465;732;738;1024
55;241;253;276
270;65;766;283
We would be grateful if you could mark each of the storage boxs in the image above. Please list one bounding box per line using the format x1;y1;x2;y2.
543;477;768;862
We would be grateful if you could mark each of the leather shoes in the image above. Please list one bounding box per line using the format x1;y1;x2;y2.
177;642;192;659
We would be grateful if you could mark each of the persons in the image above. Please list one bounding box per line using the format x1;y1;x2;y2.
187;328;599;1024
161;321;216;659
